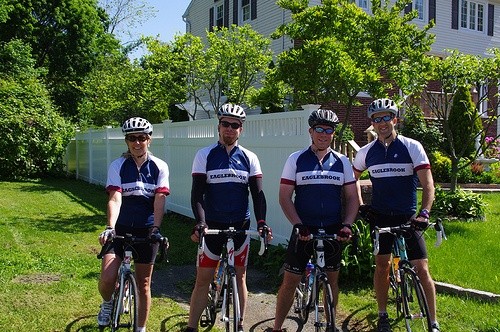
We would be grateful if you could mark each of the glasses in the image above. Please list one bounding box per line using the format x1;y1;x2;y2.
372;115;393;123
314;127;334;134
126;134;148;141
220;121;240;129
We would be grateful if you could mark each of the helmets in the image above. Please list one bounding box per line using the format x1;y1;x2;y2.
367;98;397;120
121;117;153;136
217;104;246;123
308;109;339;128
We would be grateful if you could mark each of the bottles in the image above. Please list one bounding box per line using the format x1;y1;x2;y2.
393;254;401;275
397;268;401;282
305;263;314;289
307;270;314;301
214;257;223;286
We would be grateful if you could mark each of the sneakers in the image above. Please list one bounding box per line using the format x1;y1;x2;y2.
98;297;113;325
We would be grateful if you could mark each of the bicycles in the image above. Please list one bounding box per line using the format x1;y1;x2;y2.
196;223;269;332
97;232;170;332
293;227;359;332
370;217;448;332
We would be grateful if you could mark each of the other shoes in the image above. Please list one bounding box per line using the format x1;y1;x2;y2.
378;316;391;332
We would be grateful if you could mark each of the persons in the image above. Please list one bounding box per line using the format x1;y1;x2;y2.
97;117;170;332
182;103;270;332
272;110;359;332
352;99;440;332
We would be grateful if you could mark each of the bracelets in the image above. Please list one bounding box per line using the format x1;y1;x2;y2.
257;219;264;224
